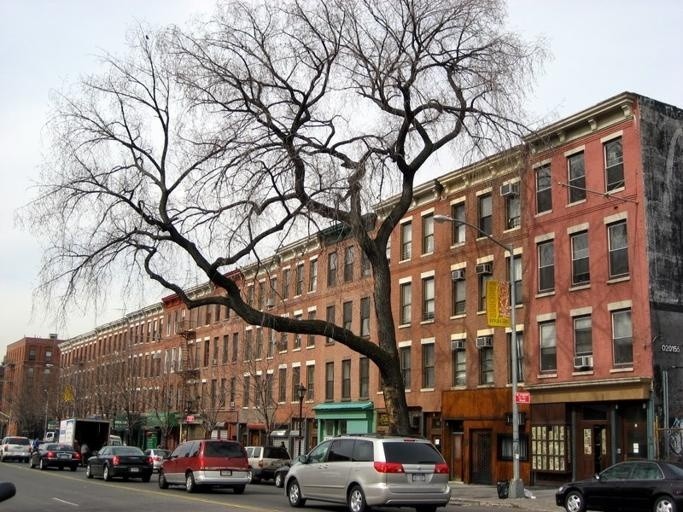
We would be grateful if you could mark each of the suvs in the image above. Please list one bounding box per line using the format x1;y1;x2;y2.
283;433;450;511
244;446;293;485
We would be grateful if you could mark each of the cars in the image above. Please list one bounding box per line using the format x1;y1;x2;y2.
157;439;253;496
86;446;154;482
145;449;174;472
29;443;81;472
1;436;33;464
274;454;312;488
556;458;682;512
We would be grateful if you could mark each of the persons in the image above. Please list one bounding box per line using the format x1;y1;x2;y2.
279;439;287;450
29;436;41;455
79;440;92;466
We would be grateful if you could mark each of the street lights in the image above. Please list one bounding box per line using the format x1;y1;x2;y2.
41;389;50;440
45;363;65;419
184;400;192;440
296;382;306;455
432;214;525;499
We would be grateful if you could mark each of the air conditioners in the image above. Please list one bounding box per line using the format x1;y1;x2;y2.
451;270;464;282
263;296;275;307
474;263;492;275
450;339;466;352
474;336;491;349
499;182;519;198
573;354;590;368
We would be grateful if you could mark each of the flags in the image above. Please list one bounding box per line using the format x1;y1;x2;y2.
483;273;513;329
63;383;74;403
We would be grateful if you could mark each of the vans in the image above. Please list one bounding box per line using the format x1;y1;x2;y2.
108;435;123;447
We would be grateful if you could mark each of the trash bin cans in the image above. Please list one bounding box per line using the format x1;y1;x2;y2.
496;479;510;498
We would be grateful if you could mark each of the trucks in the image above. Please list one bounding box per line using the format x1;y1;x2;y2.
58;419;111;467
